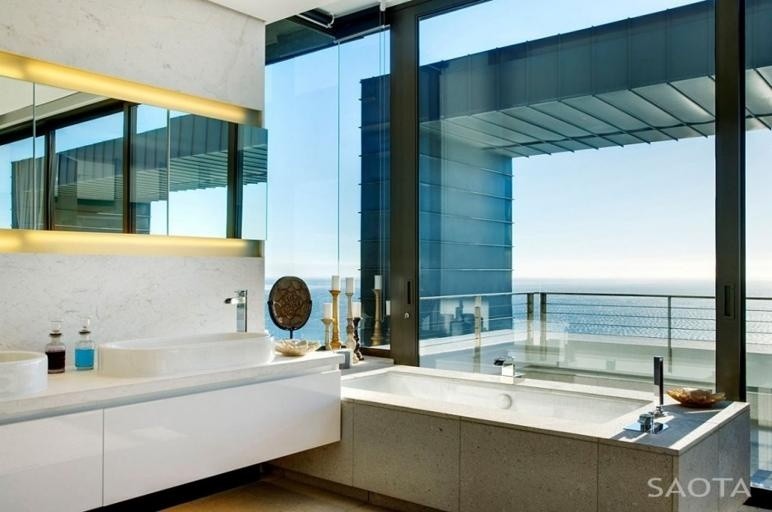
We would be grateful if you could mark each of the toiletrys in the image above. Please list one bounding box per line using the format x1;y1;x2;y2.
43;318;65;375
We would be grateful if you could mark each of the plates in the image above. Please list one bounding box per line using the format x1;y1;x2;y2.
276;339;318;357
667;385;724;407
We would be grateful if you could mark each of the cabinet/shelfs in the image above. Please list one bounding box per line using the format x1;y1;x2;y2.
0;368;343;511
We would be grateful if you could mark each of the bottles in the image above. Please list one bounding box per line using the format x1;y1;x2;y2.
44;319;68;376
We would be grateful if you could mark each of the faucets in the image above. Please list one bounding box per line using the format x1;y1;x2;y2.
652;356;664;407
225;288;249;332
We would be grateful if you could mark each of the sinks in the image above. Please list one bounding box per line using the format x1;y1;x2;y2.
97;327;273;375
0;351;49;401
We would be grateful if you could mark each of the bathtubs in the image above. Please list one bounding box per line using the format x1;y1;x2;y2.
341;364;655;447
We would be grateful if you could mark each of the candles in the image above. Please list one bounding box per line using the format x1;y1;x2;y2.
331;274;354;293
323;303;333;318
374;275;382;289
352;302;363;321
386;300;390;316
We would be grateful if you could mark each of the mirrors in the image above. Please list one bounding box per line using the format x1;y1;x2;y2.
1;50;269;259
